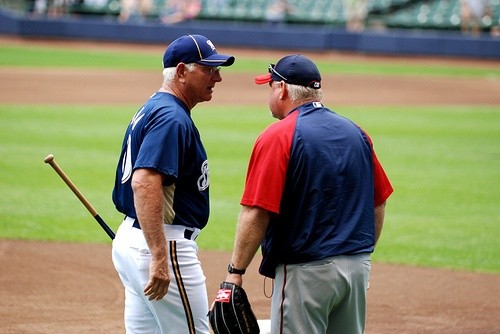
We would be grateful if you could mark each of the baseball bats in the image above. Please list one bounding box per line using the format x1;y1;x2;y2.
43;153;115;241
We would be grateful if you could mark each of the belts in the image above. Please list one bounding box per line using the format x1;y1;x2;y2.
124;215;199;241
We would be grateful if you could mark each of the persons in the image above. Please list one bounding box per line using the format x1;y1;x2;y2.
209;53;393;334
110;34;235;334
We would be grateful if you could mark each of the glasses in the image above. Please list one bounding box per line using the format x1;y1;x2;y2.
189;65;221;72
268;64;287;80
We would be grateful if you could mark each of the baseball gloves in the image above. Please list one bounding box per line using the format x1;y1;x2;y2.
206;282;260;334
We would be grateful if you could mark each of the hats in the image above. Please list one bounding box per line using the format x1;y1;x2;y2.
163;34;235;68
255;54;321;89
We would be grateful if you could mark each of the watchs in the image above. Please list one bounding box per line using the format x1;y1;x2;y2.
227;264;247;274
